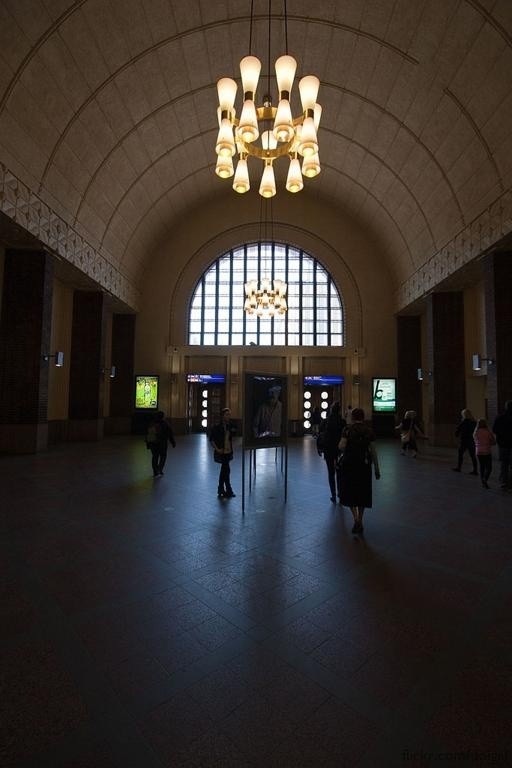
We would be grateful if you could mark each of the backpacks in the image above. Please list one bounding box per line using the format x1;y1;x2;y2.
146;423;163;449
345;429;368;462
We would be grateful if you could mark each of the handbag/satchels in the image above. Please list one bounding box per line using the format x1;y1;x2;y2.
401;432;410;442
317;432;328;452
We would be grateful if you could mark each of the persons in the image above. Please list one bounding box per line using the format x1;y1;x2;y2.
344;405;353;425
393;411;418;458
208;408;237;498
451;408;478;475
249;383;282;438
316;401;348;504
333;409;380;533
144;381;152;402
143;410;177;479
407;410;422;453
308;405;323;438
491;399;511;493
472;417;496;490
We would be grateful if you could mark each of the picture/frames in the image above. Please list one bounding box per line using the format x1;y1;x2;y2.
243;369;290;450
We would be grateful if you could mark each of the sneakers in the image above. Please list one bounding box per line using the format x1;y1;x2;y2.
400;450;417;457
481;478;488;489
218;488;235;497
352;522;363;534
453;467;477;475
154;469;164;476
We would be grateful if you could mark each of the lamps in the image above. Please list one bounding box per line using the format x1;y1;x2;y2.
472;354;493;370
42;351;65;368
215;1;323;198
101;366;116;378
417;368;432;381
244;196;287;319
